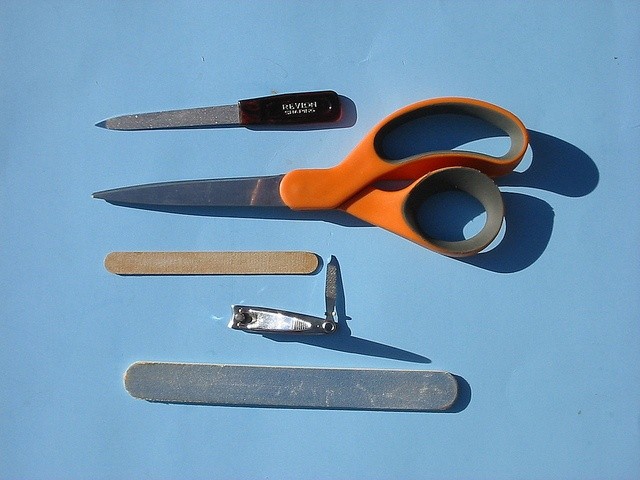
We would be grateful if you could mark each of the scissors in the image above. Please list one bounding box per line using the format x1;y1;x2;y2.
92;96;529;257
